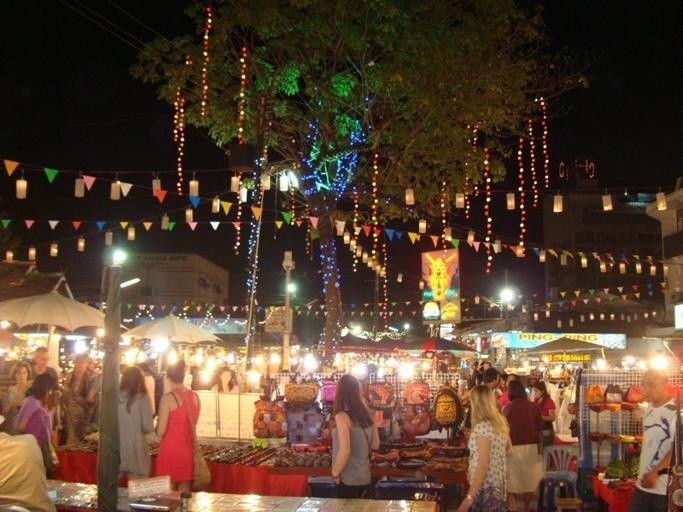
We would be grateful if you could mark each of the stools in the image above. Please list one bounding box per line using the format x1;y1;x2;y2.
555;497;584;512
537;478;577;512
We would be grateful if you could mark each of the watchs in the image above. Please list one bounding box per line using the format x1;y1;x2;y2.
466;494;475;501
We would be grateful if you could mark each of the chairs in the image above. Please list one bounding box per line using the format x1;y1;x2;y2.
544;445;580;508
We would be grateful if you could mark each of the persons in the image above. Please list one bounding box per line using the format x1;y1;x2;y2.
2;362;35;427
0;414;58;512
460;368;500;440
627;368;677;512
332;374;380;499
10;373;58;479
496;373;521;412
32;347;58;410
61;353;104;444
533;382;556;447
118;366;155;489
503;379;542;512
211;367;240;393
155;359;201;492
456;385;515;512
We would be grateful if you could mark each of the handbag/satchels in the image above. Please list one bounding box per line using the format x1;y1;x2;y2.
251;380;289;439
399;404;431;437
583;383;604;404
401;382;430;406
605;383;623;402
626;386;643;403
174;388;211;487
367;377;397;409
286;376;319;404
323;377;337;403
287;401;323;443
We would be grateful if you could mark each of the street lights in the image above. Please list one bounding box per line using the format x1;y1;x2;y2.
119;278;141;289
287;283;296;307
111;247;128;265
501;289;515;319
